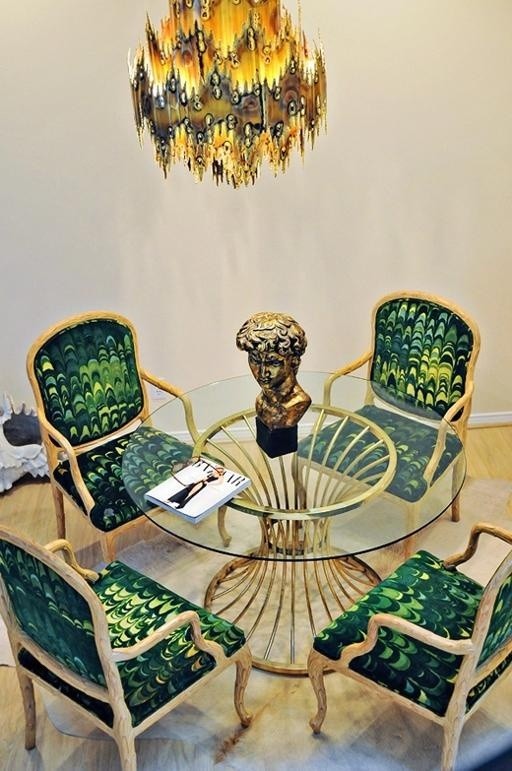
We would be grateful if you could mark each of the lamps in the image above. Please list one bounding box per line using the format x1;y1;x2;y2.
126;0;329;188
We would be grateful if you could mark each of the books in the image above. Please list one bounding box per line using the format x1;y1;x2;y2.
143;458;251;525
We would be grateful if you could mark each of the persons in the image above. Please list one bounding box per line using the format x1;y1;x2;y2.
236;312;312;431
166;467;225;509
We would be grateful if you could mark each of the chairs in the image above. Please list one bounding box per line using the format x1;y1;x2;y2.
293;294;481;556
1;535;252;771
308;523;512;771
27;311;230;564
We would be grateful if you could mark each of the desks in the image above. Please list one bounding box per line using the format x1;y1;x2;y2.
121;369;466;676
